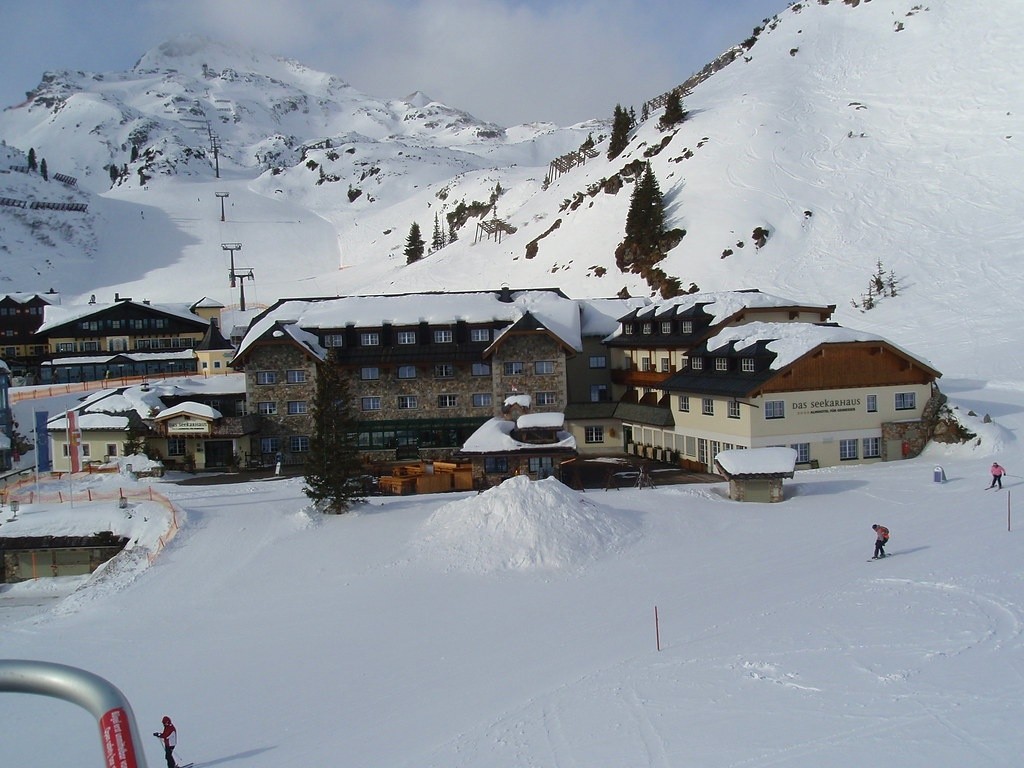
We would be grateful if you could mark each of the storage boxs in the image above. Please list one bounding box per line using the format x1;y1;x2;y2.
379;459;473;495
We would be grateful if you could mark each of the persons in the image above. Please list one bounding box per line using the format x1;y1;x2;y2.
872;524;889;559
902;439;910;459
153;716;177;768
990;462;1006;489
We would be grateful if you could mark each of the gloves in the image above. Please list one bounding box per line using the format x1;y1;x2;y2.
153;732;161;737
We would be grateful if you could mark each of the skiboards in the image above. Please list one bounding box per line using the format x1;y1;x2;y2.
177;762;196;768
866;553;891;563
985;485;1005;493
